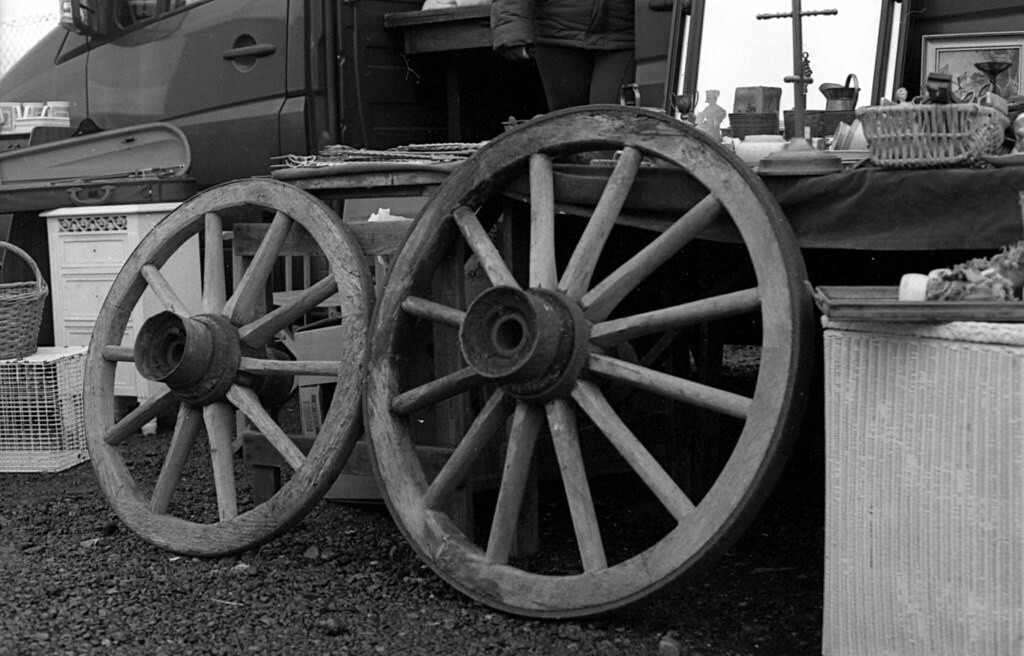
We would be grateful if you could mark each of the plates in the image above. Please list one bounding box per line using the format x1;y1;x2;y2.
980;153;1024;169
829;149;870;161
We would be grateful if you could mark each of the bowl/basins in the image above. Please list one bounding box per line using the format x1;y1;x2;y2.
727;109;874;165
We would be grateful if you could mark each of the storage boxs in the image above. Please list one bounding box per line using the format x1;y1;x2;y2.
295;312;388;507
819;312;1024;656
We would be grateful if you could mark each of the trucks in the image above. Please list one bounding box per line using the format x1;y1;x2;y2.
0;0;1024;347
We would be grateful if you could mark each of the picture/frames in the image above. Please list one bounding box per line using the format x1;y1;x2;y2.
919;30;1024;107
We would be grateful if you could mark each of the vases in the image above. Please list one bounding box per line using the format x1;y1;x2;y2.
823;109;858;137
783;109;825;141
728;113;776;141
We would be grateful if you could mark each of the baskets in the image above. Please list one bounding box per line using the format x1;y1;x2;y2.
859;105;1010;166
0;241;49;359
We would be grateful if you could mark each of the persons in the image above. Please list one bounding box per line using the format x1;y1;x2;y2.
489;0;636;114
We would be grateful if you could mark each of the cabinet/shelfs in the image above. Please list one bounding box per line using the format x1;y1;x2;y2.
38;202;228;438
